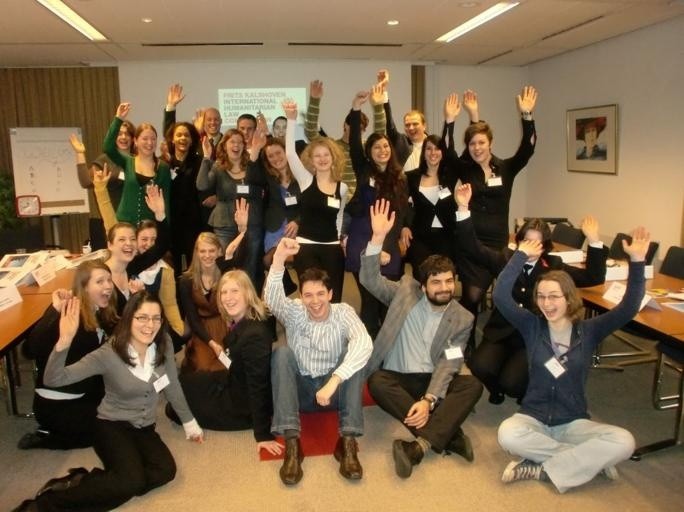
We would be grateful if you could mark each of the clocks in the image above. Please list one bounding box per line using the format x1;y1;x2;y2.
16;195;41;218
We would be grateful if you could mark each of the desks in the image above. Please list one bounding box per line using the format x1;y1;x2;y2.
574;263;684;461
507;232;609;268
17;254;88;293
0;294;58;420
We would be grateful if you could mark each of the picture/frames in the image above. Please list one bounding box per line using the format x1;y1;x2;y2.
567;103;618;174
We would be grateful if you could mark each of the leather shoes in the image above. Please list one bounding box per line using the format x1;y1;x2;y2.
515;394;525;404
487;390;508;405
333;436;363;479
279;435;304;485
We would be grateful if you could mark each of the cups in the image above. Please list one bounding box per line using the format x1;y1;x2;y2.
16;249;25;253
83;246;92;254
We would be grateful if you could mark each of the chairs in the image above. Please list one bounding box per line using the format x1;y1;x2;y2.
549;223;588;250
587;233;660;359
653;339;684;412
609;247;684;400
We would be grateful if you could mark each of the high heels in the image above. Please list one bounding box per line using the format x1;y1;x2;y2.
33;462;88;498
12;498;32;512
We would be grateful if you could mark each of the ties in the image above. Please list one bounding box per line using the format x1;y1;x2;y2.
524;263;535;283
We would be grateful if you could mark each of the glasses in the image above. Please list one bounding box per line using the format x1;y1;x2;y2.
536;294;564;303
133;315;165;325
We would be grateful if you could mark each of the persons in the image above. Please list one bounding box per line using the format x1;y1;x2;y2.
303;76;387;201
203;106;223;208
104;223;146;318
491;226;652;493
359;198;483;479
236;114;259;159
244;124;309;271
454;182;610;406
197;127;256;259
127;219;190;345
344;91;407;335
378;69;434;194
164;232;286;456
159;83;204;162
283;99;348;304
402;136;460;286
69;120;137;253
177;196;249;371
258;112;289;144
575;116;611;162
102;103;171;234
263;236;374;485
439;89;483;165
452;84;537;313
18;259;120;452
166;121;201;276
12;289;205;512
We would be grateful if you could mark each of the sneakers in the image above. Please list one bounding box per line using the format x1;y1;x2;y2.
444;424;473;466
17;423;51;450
500;455;547;483
391;436;424;479
599;464;621;481
